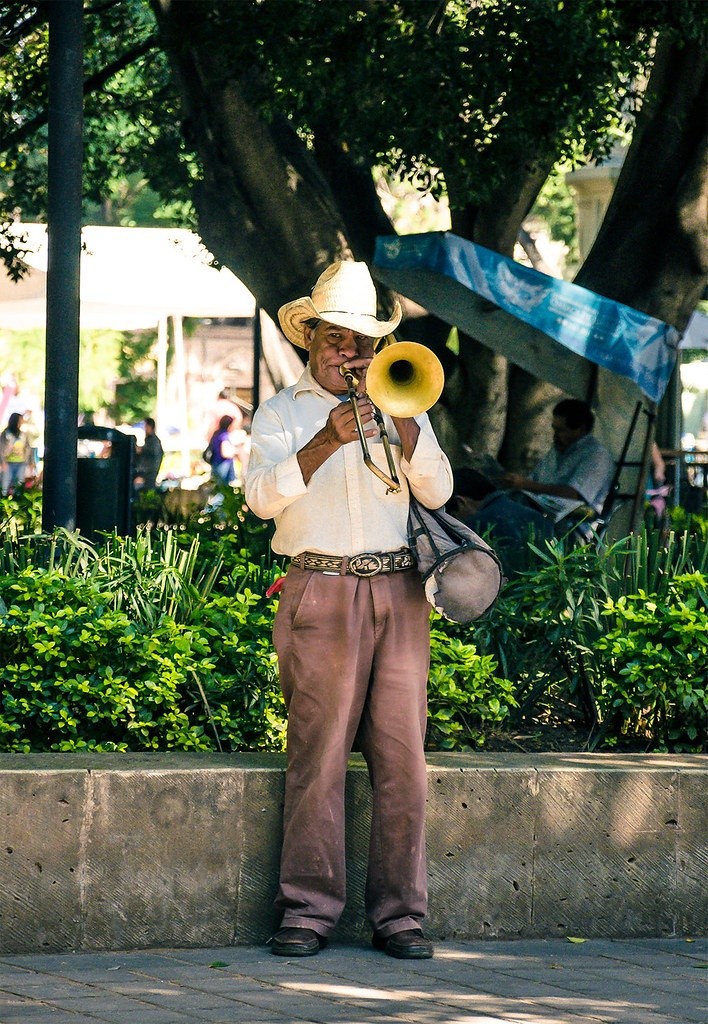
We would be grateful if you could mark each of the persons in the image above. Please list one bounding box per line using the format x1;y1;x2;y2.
133;417;164;491
207;387;242;485
244;260;455;958
0;407;44;494
445;400;617;580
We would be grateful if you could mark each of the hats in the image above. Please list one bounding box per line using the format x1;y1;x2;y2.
278;260;403;349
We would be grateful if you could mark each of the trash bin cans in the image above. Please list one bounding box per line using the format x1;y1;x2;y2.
76;424;137;545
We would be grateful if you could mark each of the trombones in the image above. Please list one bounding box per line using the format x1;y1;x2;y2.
308;221;444;495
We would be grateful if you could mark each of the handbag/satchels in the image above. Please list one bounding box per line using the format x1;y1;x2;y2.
407;478;502;624
203;443;214;464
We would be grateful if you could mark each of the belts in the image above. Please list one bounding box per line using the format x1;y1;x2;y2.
291;553;414;577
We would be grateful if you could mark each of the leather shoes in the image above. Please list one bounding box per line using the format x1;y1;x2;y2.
266;926;320;955
373;928;434;959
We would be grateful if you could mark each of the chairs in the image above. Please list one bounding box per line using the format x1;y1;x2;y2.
589;480;620;549
